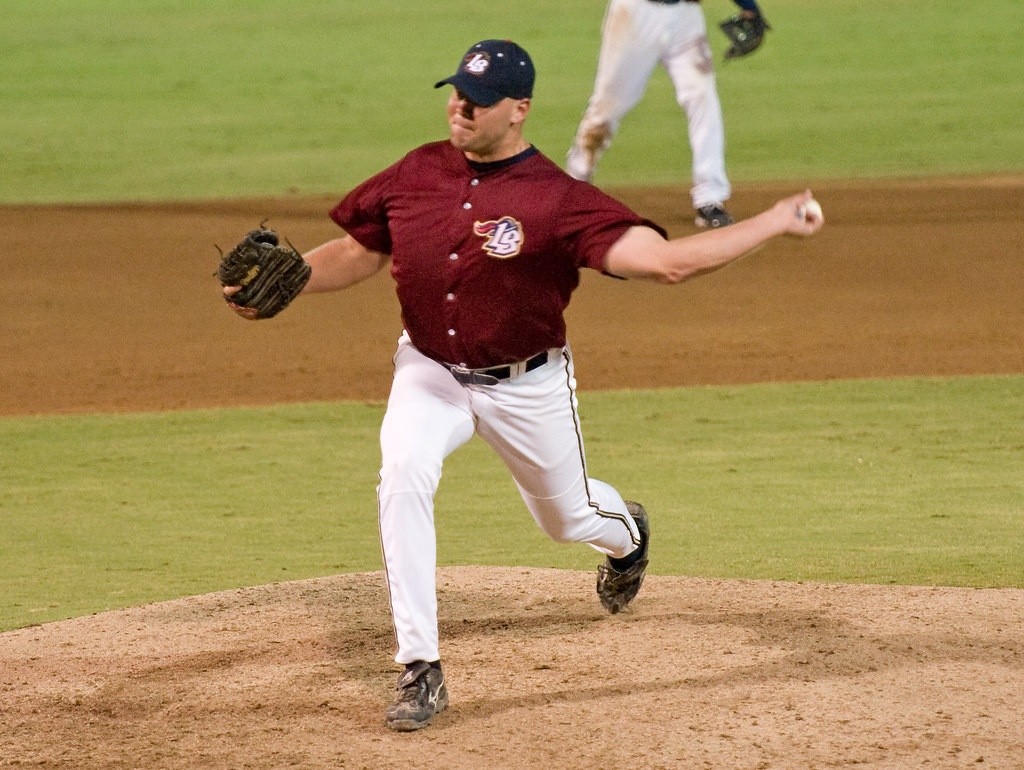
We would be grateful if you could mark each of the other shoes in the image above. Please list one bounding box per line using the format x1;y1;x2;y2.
694;204;731;228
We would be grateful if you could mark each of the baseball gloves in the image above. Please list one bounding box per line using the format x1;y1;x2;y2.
718;12;771;61
218;228;313;321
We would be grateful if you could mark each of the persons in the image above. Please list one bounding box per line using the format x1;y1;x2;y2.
222;39;824;731
565;0;773;231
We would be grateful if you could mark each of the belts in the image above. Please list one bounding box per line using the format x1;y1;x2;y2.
442;351;548;386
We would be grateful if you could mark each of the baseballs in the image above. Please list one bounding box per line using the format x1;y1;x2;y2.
803;200;824;221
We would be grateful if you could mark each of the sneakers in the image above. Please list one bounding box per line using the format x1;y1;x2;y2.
597;501;650;615
386;661;448;730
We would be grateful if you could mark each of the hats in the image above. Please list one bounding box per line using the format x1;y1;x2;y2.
435;40;535;107
